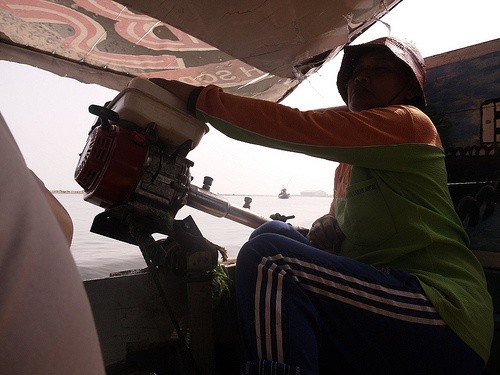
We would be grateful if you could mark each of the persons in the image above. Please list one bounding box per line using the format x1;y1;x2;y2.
147;37;496;374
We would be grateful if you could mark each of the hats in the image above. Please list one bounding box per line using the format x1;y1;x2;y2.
337;35;428;112
